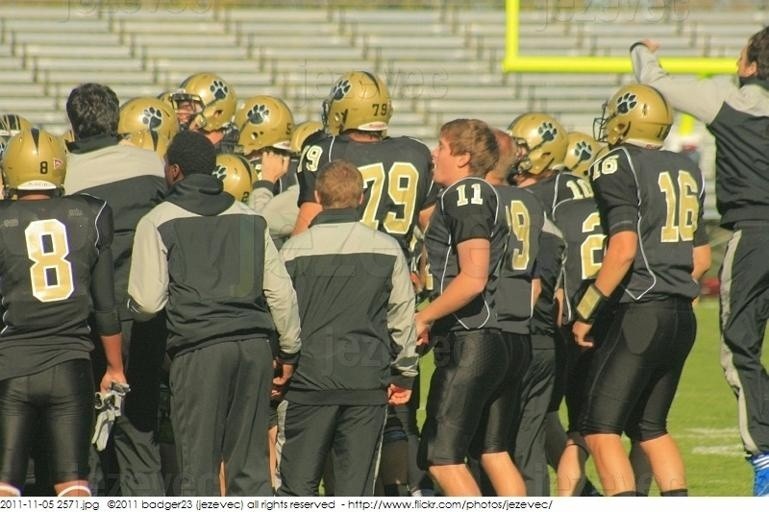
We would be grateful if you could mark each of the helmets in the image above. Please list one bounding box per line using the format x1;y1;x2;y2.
507;111;603;180
1;71;322;215
324;68;393;137
597;81;674;150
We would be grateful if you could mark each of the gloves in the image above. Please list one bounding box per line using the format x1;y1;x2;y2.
90;378;131;451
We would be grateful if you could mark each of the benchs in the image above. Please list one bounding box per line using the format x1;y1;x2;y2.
0;0;769;159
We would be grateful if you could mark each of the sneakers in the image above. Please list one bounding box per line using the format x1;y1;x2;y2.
752;462;769;496
